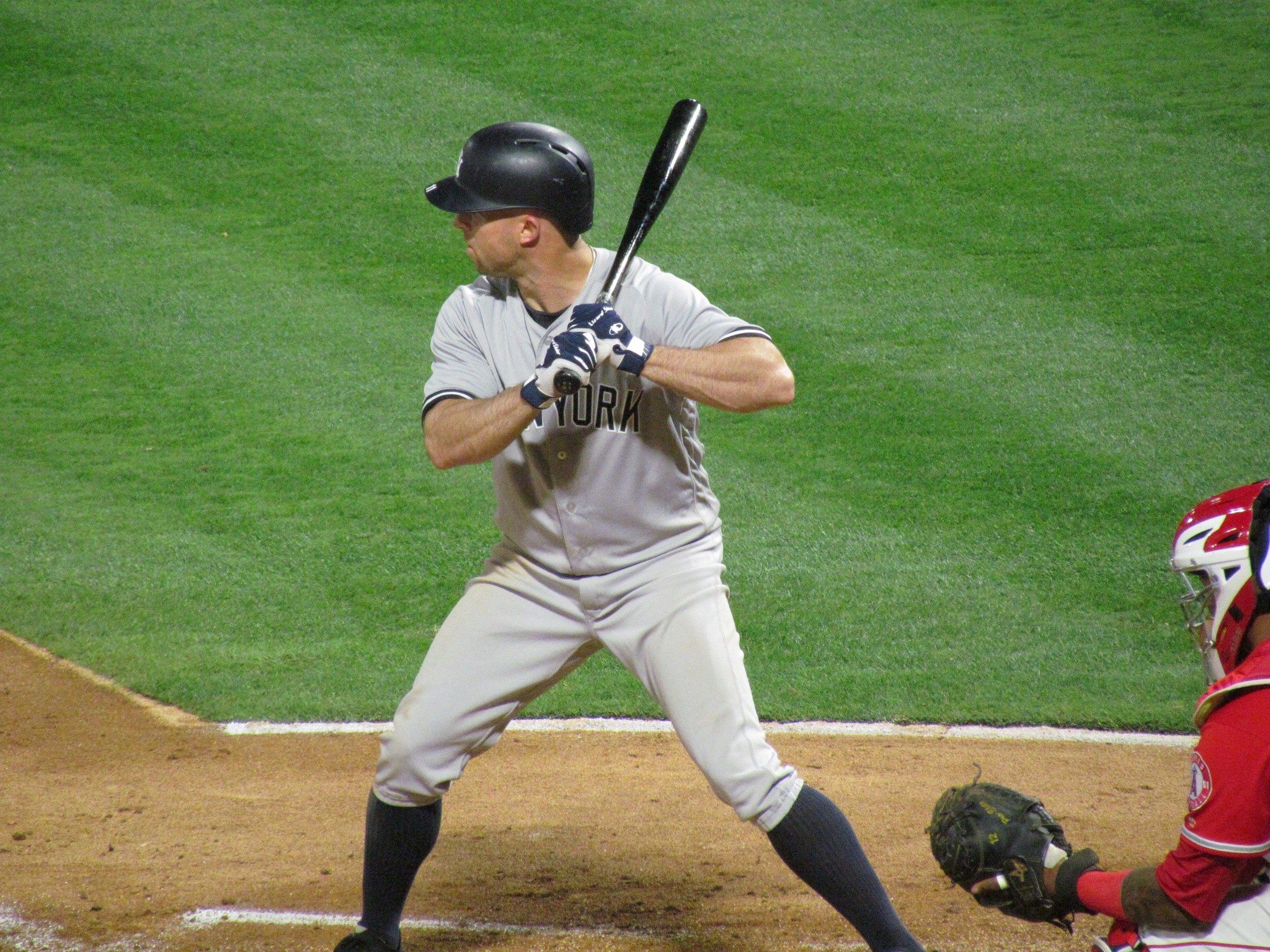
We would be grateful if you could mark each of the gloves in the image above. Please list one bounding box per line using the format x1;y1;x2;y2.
519;328;600;409
567;303;654;378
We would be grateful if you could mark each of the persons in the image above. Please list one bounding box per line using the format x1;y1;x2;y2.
334;119;927;952
922;479;1270;952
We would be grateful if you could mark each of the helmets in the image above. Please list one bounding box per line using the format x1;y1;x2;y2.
424;121;596;237
1170;477;1270;685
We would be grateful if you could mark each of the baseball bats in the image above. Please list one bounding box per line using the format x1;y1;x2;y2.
553;98;708;395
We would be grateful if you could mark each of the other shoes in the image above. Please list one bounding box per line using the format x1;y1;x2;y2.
333;923;402;952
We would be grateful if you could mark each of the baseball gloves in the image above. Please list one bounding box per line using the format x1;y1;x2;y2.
929;783;1108;923
